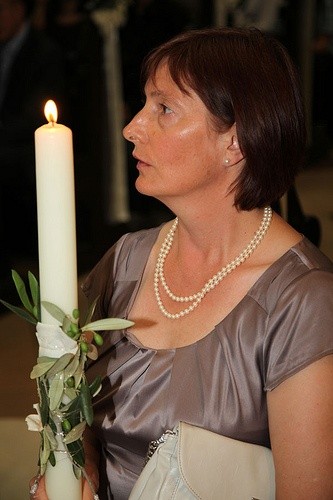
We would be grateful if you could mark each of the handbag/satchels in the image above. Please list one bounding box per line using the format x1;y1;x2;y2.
128;420;275;500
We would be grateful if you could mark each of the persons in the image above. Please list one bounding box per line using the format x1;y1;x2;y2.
30;25;333;500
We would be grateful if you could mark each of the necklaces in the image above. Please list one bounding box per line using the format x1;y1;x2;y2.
153;206;272;318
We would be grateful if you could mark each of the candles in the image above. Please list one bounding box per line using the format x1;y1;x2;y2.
34;123;83;500
